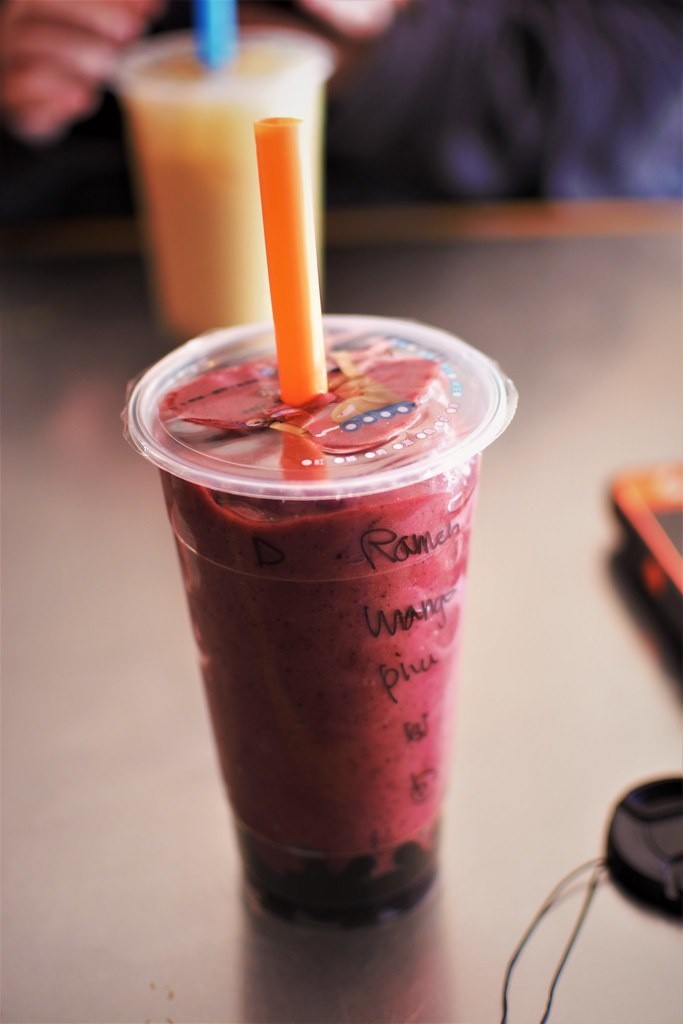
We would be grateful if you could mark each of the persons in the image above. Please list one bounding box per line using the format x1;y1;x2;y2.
0;0;683;210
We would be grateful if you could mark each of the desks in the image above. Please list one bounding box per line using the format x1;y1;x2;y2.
0;200;683;1024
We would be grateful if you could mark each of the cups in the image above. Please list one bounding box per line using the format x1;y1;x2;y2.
124;315;518;927
113;27;338;345
